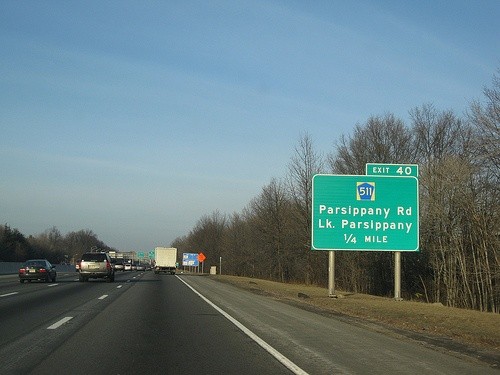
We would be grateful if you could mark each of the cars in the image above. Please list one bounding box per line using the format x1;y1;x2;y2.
76;261;79;272
18;259;56;283
114;262;154;272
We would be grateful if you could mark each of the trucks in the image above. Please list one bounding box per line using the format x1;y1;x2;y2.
154;247;177;275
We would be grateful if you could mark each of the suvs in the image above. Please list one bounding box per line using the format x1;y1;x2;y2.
78;252;116;283
